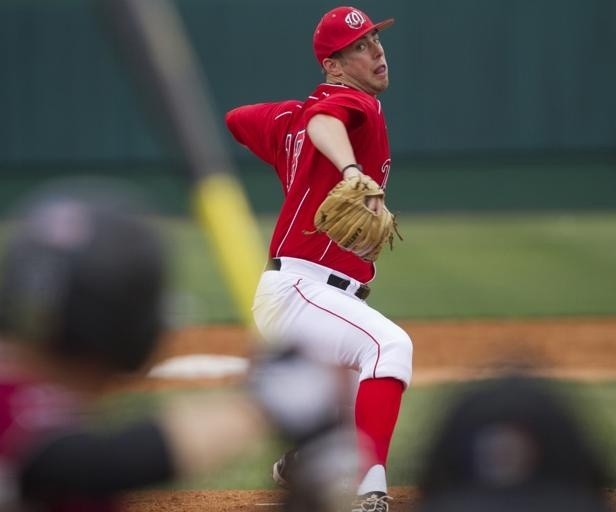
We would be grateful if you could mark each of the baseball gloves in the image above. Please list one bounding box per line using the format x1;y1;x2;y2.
302;173;403;262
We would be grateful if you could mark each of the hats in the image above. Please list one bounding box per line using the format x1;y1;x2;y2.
314;6;394;65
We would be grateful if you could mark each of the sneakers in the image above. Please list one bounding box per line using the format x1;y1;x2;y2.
274;447;388;512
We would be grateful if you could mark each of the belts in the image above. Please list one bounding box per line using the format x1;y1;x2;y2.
265;258;371;300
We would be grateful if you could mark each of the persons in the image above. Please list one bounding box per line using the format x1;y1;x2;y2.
223;5;413;512
